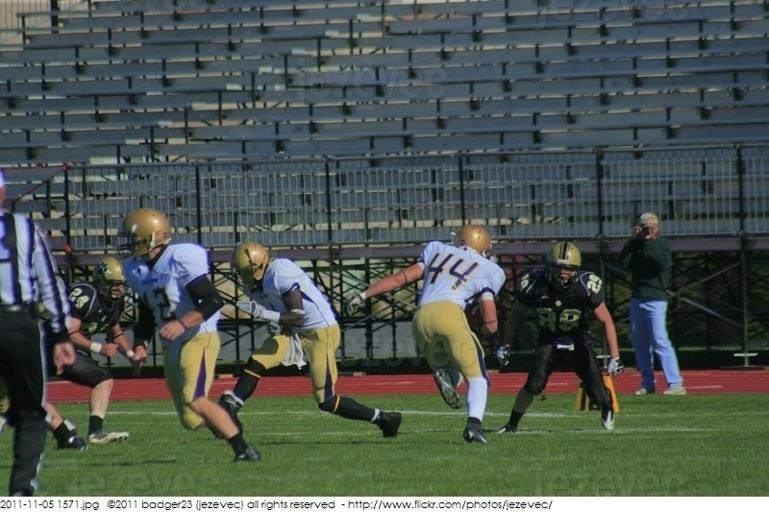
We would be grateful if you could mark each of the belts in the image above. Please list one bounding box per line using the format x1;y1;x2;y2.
0;304;33;313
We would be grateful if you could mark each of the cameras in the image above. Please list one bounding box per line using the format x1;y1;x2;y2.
638;226;650;238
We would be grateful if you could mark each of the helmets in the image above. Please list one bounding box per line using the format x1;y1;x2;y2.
231;241;268;296
545;241;581;269
116;208;171;261
93;258;130;306
455;224;492;256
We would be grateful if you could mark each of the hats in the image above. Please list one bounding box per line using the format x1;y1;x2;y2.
638;213;659;225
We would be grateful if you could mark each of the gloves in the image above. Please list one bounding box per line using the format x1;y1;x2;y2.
236;300;263;320
343;294;366;317
496;348;513;370
608;357;623;377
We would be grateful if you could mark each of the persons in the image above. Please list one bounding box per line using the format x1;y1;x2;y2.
216;243;403;437
497;241;624;434
345;227;505;442
42;259;142;445
116;209;260;466
620;213;689;398
1;388;87;453
0;168;76;497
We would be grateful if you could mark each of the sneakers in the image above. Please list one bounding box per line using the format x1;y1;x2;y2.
62;438;90;453
635;386;655;396
601;406;615;431
235;445;262;463
380;411;402;438
436;372;462;410
498;426;517;434
87;431;130;445
203;399;243;439
462;425;489;445
664;388;687;396
